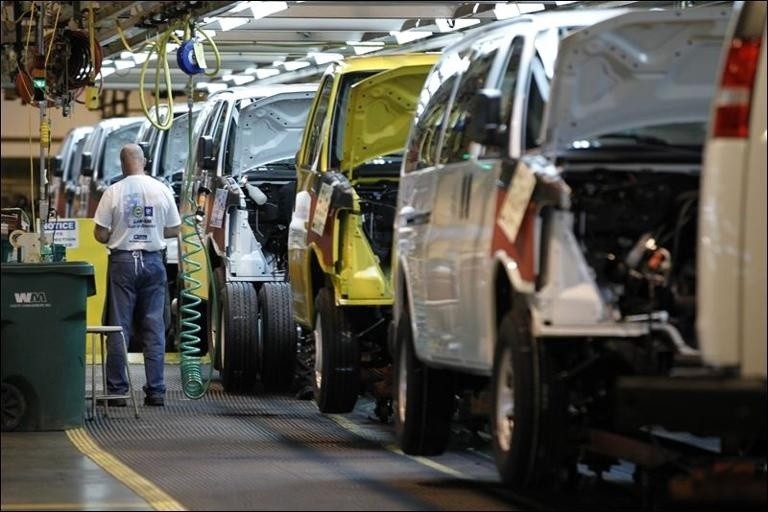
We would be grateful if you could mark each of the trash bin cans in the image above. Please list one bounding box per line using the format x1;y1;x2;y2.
0;260;97;433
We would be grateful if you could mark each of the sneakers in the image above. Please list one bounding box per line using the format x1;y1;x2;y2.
96;398;127;406
144;395;164;405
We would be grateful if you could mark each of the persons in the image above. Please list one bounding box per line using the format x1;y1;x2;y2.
91;142;182;408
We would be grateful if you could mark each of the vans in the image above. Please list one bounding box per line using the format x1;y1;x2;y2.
695;1;768;387
381;0;740;502
287;49;446;409
182;84;332;395
45;101;203;356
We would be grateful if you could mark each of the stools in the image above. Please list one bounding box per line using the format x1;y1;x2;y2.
84;324;140;421
